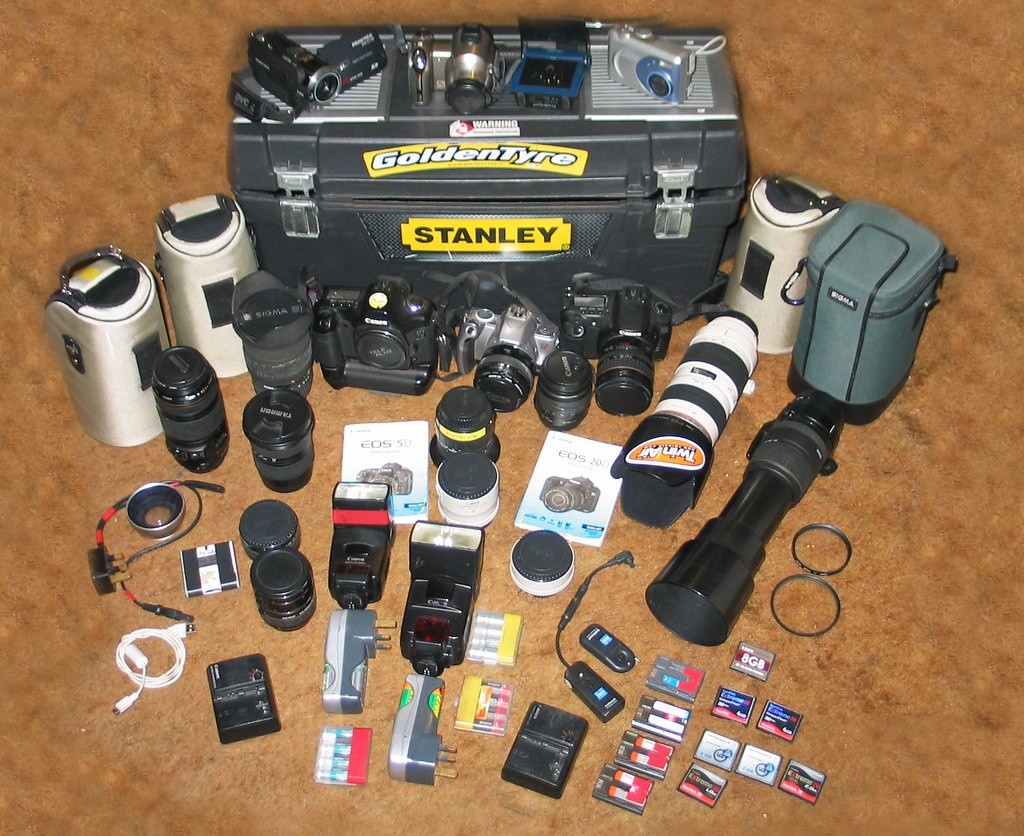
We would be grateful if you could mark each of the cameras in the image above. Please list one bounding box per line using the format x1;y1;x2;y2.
455;305;560;413
560;285;674;418
246;25;390;115
314;274;447;397
606;20;697;103
